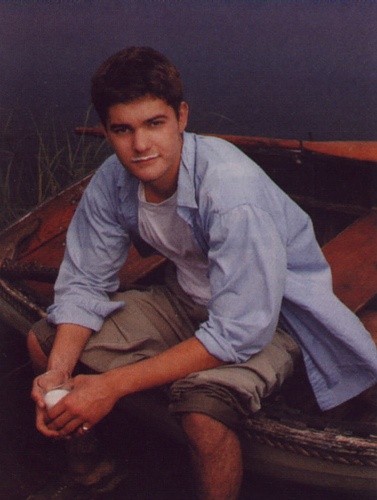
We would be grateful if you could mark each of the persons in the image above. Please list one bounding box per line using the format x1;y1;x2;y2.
26;47;332;499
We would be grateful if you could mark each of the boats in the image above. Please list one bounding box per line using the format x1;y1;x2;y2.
0;133;377;500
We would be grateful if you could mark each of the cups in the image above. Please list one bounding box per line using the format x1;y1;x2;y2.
36;370;74;421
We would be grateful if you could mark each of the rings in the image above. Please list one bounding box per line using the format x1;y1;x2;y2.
82;426;89;430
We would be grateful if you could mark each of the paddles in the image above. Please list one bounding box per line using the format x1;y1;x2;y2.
75;123;377;162
